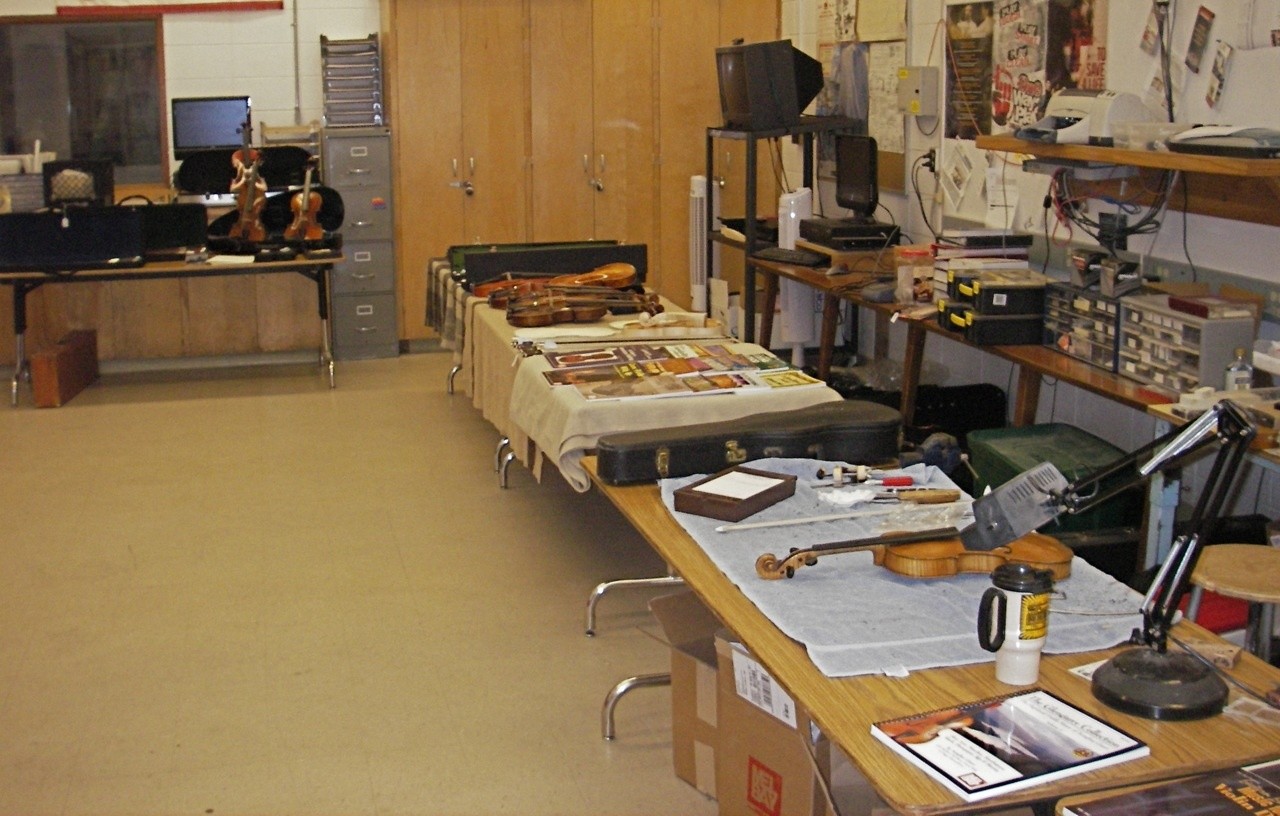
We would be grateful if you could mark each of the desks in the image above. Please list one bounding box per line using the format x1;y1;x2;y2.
423;252;1280;816
0;256;346;408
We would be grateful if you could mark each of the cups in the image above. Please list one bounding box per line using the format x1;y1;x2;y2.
978;564;1055;686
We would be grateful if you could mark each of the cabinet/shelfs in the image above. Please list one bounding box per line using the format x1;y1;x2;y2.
319;0;865;361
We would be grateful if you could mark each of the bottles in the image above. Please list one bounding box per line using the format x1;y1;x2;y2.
1225;348;1252;392
894;250;935;303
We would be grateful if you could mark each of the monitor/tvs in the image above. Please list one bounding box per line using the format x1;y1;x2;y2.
833;132;878;225
712;38;825;130
171;96;250;158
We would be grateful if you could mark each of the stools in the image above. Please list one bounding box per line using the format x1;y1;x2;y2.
1186;543;1280;665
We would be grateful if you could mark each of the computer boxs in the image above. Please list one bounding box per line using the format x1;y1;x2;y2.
800;216;900;250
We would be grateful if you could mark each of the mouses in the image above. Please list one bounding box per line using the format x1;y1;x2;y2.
825;262;849;274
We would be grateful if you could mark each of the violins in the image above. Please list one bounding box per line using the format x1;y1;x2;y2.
228;120;269;193
752;523;1076;584
282;156;325;241
228;149;269;242
466;262;665;328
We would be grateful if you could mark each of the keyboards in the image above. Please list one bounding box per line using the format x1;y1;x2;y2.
750;246;829;266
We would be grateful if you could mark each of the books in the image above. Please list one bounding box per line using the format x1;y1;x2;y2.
870;686;1150;804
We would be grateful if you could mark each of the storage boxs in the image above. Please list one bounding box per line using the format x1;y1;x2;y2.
646;589;831;816
966;422;1136;566
